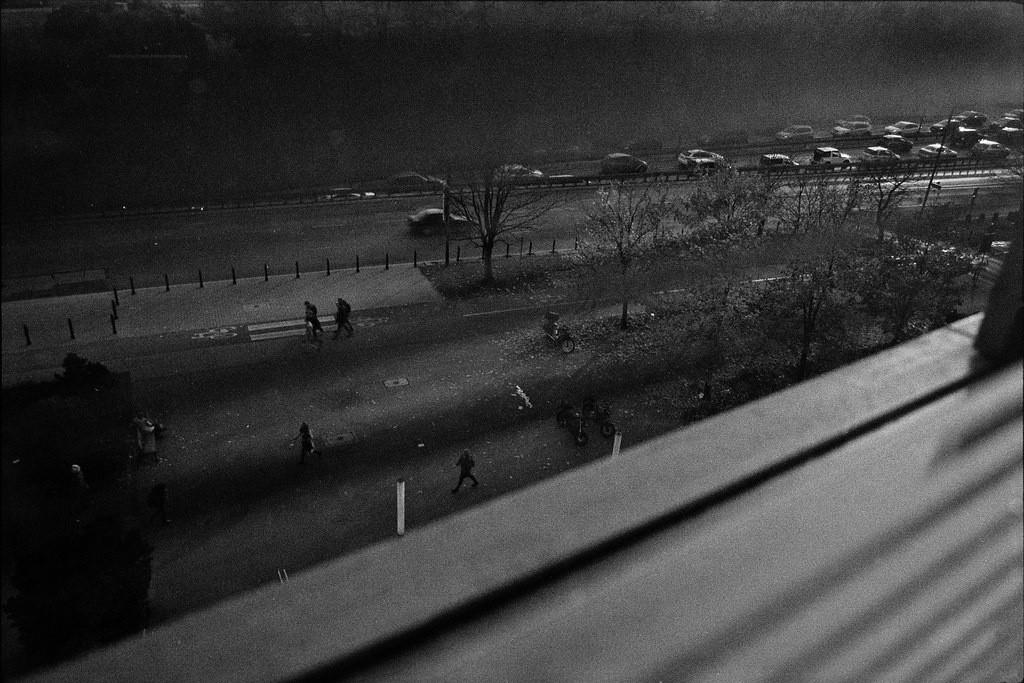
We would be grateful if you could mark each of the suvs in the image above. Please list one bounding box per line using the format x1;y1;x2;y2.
878;134;913;155
810;146;852;171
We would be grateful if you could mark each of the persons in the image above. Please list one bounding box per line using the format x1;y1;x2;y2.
70;463;94;523
305;301;324;338
449;448;478;493
333;297;353;339
293;420;323;465
151;483;173;524
303;318;318;352
123;526;154;562
137;417;158;465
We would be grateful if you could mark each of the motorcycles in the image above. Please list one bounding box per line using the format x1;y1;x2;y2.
556;395;616;446
542;309;576;355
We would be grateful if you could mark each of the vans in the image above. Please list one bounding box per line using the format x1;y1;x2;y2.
757;154;801;176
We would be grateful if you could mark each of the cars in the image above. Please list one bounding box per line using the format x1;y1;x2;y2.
775;124;815;145
885;121;919;137
830;114;873;141
918;108;1024;161
302;165;543;201
601;153;650;175
678;149;724;171
406;208;476;237
860;145;901;165
